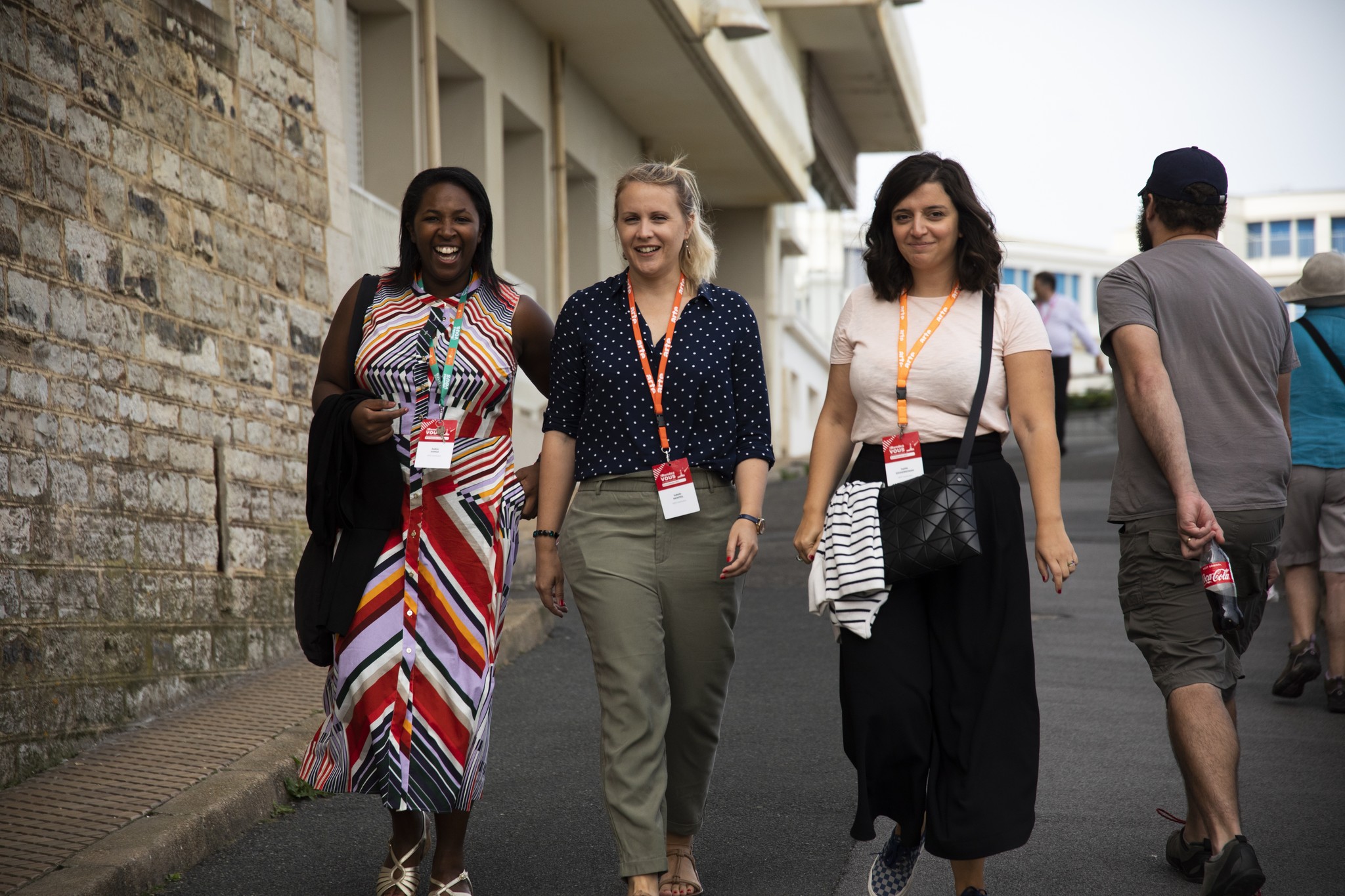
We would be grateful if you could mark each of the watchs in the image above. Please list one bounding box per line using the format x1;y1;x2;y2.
736;514;766;535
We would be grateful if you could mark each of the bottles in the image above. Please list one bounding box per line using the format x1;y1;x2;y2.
1203;539;1244;636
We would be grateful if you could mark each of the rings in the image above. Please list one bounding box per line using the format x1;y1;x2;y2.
795;555;804;561
1067;560;1075;566
1185;537;1193;545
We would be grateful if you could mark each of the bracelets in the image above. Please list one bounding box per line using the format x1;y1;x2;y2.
532;529;559;538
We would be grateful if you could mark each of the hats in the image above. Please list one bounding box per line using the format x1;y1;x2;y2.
1138;146;1228;205
1278;252;1345;307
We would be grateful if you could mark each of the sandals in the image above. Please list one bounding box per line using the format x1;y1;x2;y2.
428;869;473;896
658;845;703;896
376;810;431;896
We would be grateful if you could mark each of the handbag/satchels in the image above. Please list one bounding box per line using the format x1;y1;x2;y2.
877;464;983;582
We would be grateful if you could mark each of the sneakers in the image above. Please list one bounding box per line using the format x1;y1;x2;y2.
868;825;925;896
1272;634;1322;698
1197;835;1266;896
1324;670;1345;713
1157;809;1212;884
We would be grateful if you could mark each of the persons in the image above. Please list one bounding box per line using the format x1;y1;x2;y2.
534;162;777;896
791;150;1079;896
297;163;572;896
1272;251;1345;715
1030;271;1108;454
1095;146;1305;896
